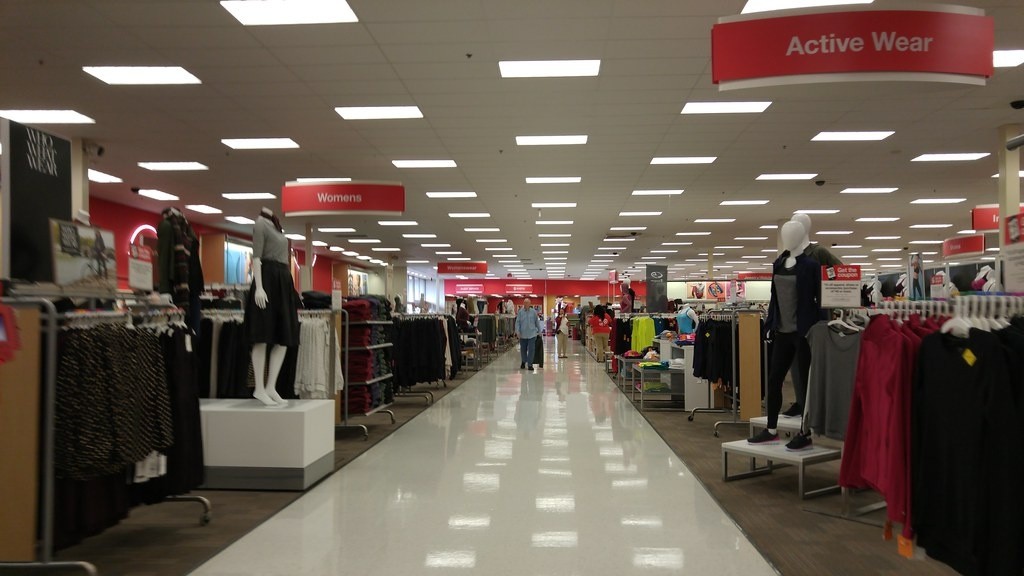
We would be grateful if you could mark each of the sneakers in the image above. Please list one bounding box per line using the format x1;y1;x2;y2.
785;433;813;452
747;428;779;446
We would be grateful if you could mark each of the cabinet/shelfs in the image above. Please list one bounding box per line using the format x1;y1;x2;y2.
342;320;397;425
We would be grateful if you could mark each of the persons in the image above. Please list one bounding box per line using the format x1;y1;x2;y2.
780;214;844;418
497;300;506;314
623;279;635;311
748;221;820;452
588;305;613;363
936;271;960;298
158;206;204;340
456;303;469;330
619;283;631;312
94;231;107;279
555;309;570;358
972;266;1002;292
514;371;538;429
691;283;705;298
81;247;94;279
515;299;542;370
866;277;883;303
245;206;299;406
476;299;487;314
911;254;922;300
577;304;582;312
677;304;699;334
556;358;568;402
896;274;908;296
507;300;514;315
456;297;463;312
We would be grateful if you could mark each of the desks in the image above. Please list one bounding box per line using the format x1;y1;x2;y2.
652;338;709;411
632;364;684;411
748;414;803;470
618;358;660;393
721;437;842;500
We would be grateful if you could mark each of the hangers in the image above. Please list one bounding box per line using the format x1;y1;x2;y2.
829;308;882;331
393;313;449;321
883;299;950;323
617;312;677;319
62;309;188;332
699;314;733;322
202;310;331;322
942;292;1024;335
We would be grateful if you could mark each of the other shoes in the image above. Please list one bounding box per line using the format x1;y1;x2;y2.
563;355;568;358
782;401;801;417
558;355;562;358
521;361;525;369
528;366;534;370
